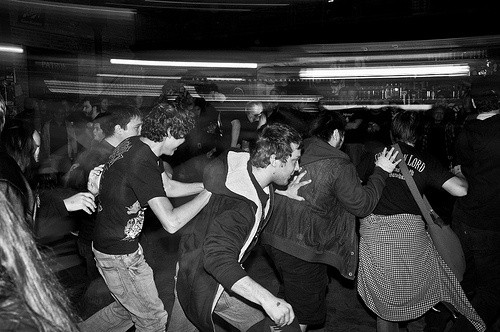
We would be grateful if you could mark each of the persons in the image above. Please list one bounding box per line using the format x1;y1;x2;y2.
0;73;500;332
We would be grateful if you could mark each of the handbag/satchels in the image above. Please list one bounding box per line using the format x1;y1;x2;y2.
426;217;467;285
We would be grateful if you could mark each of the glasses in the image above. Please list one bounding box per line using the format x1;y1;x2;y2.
253;111;264;118
